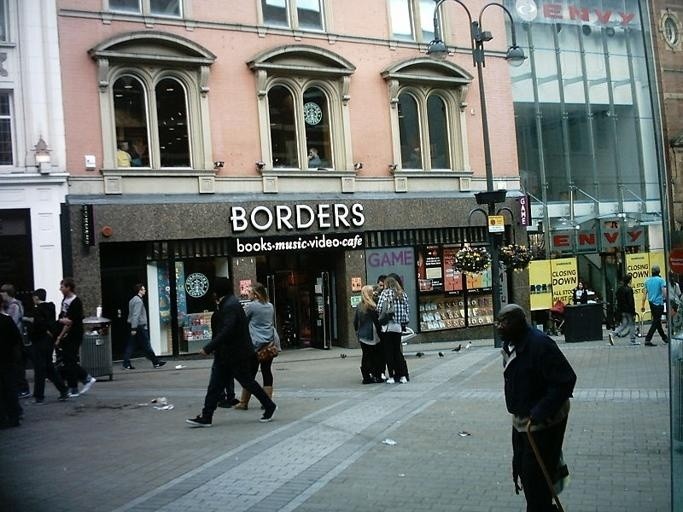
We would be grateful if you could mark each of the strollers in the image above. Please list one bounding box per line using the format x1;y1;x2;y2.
545;300;570;336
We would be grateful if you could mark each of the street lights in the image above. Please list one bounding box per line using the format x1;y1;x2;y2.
425;0;528;349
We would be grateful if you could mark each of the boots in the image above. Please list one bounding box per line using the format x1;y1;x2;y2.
258;385;273;410
234;385;251;411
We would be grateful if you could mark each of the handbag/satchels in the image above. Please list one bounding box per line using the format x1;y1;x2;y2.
256;341;279;363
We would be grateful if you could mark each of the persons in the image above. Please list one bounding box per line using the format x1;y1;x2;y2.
0;279;96;431
667;271;681;317
208;292;238;409
116;144;132;167
120;140;138;161
494;303;578;512
122;282;167;371
185;276;278;428
572;281;587;305
306;147;322;169
608;274;641;346
236;282;277;411
352;272;410;384
641;264;670;346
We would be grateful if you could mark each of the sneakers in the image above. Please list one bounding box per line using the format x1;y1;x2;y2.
400;376;407;383
259;405;278;423
18;391;45;406
631;340;641;345
386;377;395;384
57;392;79;401
153;361;167;368
79;374;97;395
662;338;670;346
120;361;135;370
608;332;614;346
185;415;213;428
643;341;657;346
362;376;389;385
216;398;240;408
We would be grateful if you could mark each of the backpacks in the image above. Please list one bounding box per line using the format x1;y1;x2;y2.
20;317;32;354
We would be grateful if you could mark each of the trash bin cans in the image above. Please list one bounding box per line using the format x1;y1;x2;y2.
565;303;603;343
80;316;114;377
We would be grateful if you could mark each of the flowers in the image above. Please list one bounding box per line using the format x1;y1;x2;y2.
453;244;491;279
497;244;532;275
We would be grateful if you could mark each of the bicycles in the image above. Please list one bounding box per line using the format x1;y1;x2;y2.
590;295;633;339
